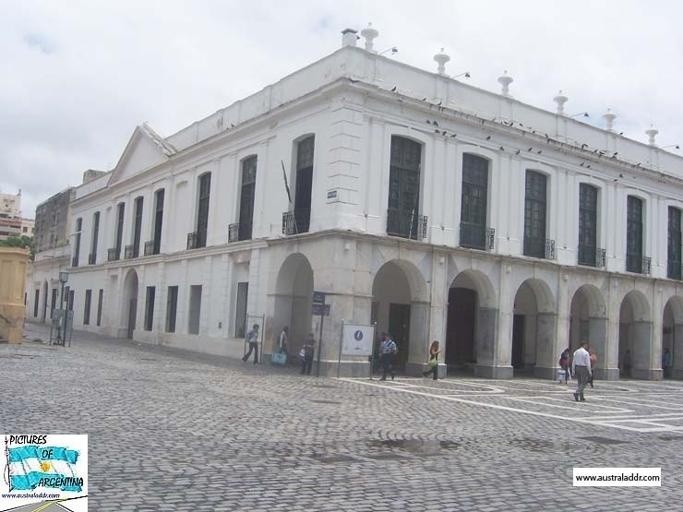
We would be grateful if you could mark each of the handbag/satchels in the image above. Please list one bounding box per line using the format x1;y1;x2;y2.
272;349;288;367
426;358;437;369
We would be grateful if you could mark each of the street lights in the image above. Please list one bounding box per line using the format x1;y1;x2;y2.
53;270;69;346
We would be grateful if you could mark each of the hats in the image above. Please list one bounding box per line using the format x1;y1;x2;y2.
578;340;587;346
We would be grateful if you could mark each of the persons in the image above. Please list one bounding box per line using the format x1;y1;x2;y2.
622;349;631;377
662;347;671;379
241;324;259;366
422;340;441;379
279;326;288;352
378;333;397;381
587;347;597;388
571;339;592;401
298;333;315;374
558;348;570;384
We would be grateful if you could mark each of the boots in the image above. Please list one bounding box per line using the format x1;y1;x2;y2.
573;393;586;402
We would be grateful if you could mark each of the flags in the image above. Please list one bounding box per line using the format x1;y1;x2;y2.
6;445;82;493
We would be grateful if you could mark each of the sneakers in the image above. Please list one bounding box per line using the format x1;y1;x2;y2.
422;371;440;381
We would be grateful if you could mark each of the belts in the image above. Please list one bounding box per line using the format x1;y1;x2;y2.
575;364;586;368
